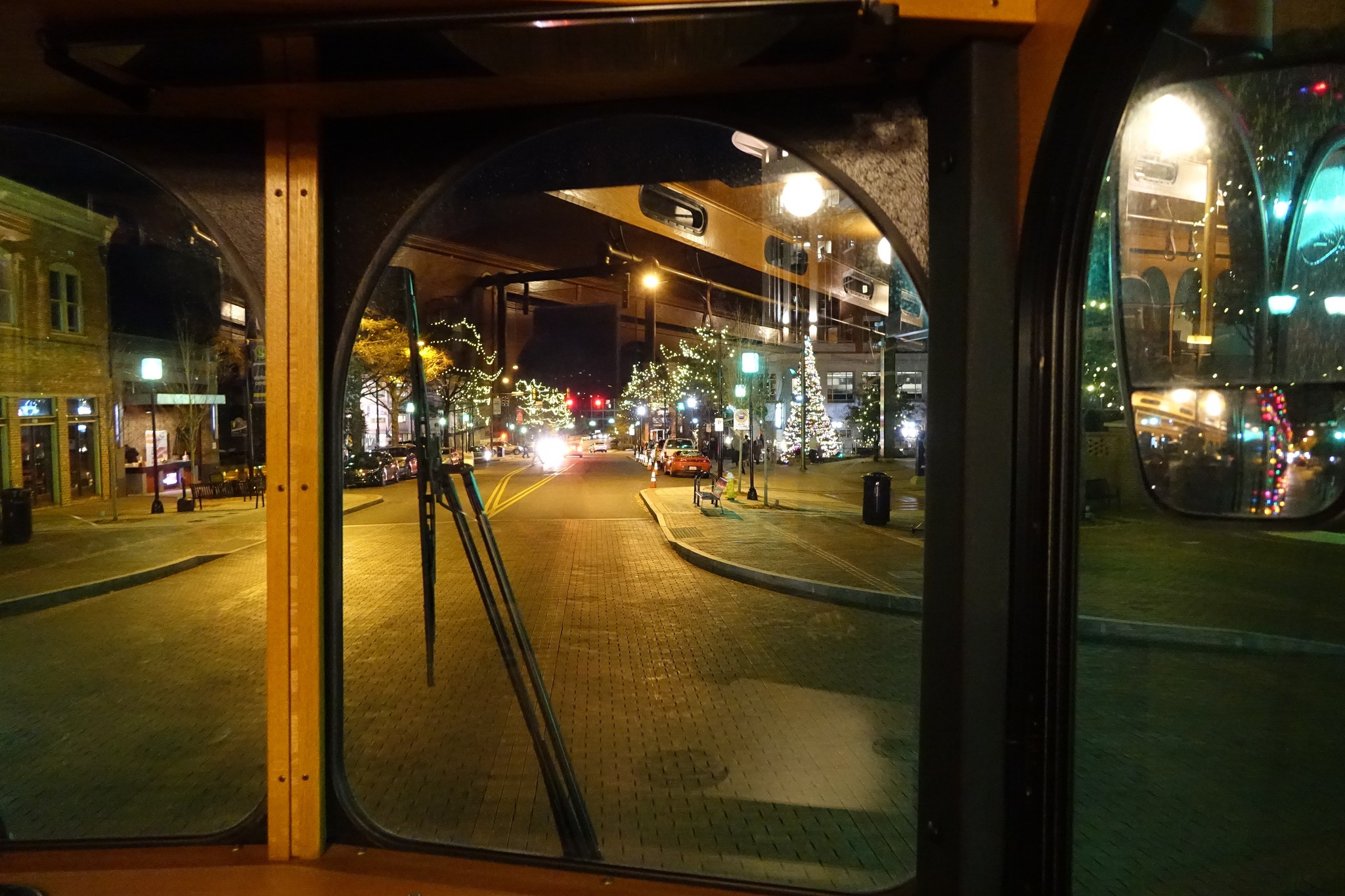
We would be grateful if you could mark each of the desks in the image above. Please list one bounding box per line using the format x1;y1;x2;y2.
125;459;199;494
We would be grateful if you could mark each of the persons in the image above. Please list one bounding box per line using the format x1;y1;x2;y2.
743;434;750;467
645;442;648;450
753;436;763;465
124;444;139;464
522;442;530;458
732;434;743;467
809;436;820;464
531;440;545;467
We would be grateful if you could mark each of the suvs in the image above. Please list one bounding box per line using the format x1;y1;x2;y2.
376;442;418;480
485;441;524;456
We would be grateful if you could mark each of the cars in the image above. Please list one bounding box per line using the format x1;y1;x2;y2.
589;442;607;453
581;432;609;440
1138;425;1341;508
440;447;461;466
563;443;583;458
467;445;492;463
343;450;401;489
645;438;712;478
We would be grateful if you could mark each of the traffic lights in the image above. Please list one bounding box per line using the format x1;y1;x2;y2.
595;400;601;406
567;400;572;405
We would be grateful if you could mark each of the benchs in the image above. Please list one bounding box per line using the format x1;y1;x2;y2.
694;477;728;515
190;475;266;510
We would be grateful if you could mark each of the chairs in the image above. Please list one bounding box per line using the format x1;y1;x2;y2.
1122;275;1164;383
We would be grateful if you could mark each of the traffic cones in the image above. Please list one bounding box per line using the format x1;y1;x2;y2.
652;461;659;476
635;452;648;466
648;469;657;489
647;457;653;470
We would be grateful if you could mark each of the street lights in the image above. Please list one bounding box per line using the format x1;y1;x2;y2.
742;351;759;501
735;384;746;406
490;377;509;450
464;414;470;452
638;406;645;452
406;402;415;441
140;357;166;513
440;417;446;450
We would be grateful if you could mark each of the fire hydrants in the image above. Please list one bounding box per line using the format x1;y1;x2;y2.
724;473;736;500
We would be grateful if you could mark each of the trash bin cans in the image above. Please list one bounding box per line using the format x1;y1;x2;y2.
860;472;892;525
2;488;35;545
